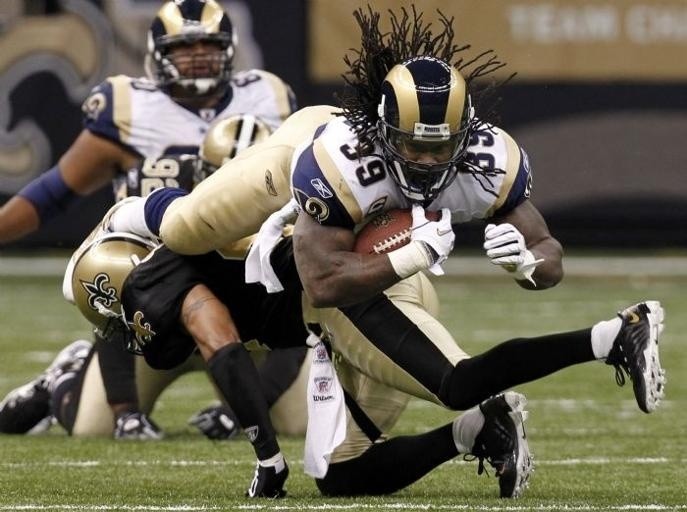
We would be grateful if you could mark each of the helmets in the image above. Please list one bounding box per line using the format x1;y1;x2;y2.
193;112;273;185
145;0;240;105
375;56;475;213
71;232;158;356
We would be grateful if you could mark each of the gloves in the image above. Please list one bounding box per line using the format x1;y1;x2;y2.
112;407;165;439
483;222;545;288
245;452;289;498
187;400;241;439
409;203;456;277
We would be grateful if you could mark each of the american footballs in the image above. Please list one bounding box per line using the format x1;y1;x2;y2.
352;210;443;254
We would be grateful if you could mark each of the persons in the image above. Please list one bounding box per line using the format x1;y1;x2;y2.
63;3;669;502
0;0;312;436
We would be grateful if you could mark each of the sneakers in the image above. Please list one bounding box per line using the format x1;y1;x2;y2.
604;300;667;413
463;391;534;500
2;341;92;435
62;195;143;307
